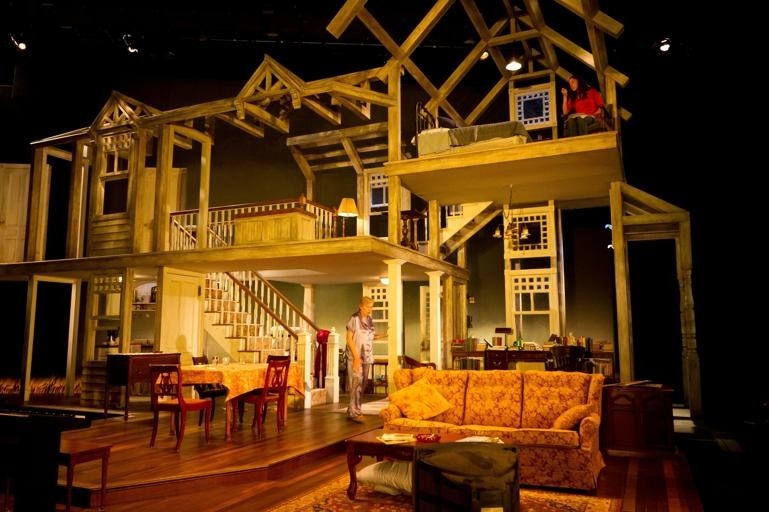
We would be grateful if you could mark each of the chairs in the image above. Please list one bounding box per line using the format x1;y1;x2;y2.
398;354;436;370
147;363;212;453
545;346;593;374
561;93;612;137
191;357;243;426
229;354;291;439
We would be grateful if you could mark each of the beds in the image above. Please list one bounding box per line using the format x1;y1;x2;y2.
410;102;532;157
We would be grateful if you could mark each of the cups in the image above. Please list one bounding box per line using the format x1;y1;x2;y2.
221;356;231;369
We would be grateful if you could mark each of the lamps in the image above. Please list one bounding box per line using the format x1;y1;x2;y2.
8;32;28;52
120;31;140;54
380;277;389;286
657;34;672;54
337;198;359;237
493;183;531;242
505;48;522;72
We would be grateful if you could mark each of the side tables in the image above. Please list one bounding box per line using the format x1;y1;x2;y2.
603;447;692;512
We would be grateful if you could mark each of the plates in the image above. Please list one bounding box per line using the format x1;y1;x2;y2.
416;432;442;443
379;431;414;443
372;435;417;446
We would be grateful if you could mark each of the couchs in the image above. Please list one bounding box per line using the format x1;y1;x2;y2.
378;368;607;495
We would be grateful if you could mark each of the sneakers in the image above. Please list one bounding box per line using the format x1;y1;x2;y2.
347;411;368;423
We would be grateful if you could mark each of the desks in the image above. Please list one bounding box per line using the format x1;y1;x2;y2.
154;363;305;441
106;352;181;421
451;350;615;384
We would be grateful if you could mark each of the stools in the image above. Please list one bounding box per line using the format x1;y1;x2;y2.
58;438;112;511
372;359;388;394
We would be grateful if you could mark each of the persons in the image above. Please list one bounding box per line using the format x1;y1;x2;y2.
562;75;603;121
346;296;375;423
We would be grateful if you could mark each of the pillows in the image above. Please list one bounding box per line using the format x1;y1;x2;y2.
355;459;412;495
387;370;595;430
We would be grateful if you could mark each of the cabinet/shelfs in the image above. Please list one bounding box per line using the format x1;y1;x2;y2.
601;384;673;451
82;267;159;411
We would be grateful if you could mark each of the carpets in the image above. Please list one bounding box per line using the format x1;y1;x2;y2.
258;468;624;512
